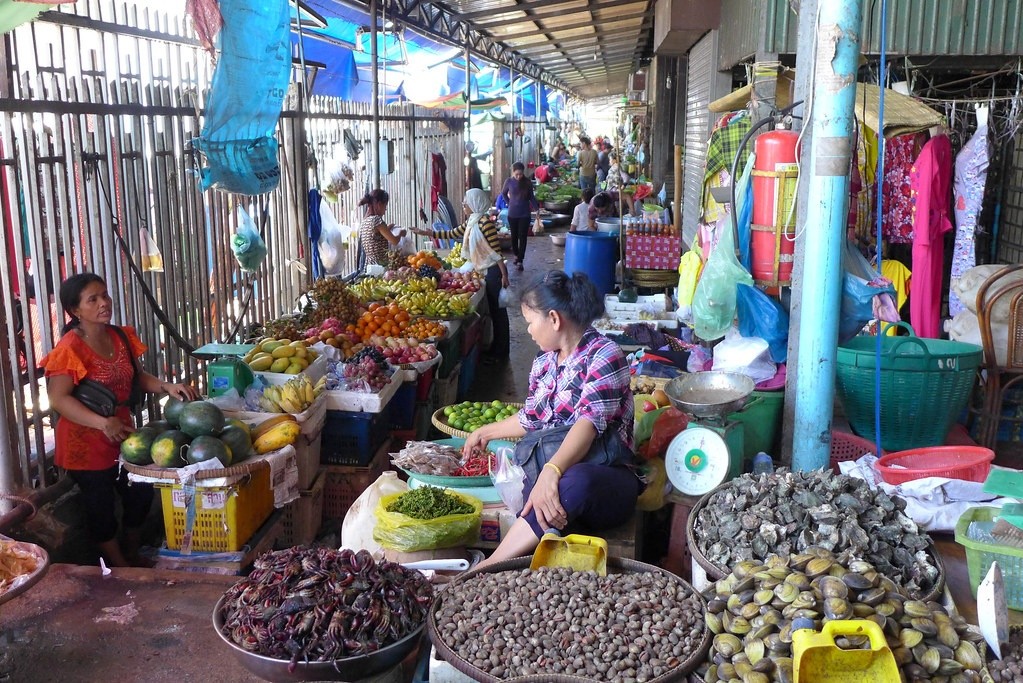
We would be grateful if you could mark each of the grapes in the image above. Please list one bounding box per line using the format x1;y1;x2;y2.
419;264;441;282
340;345;393;391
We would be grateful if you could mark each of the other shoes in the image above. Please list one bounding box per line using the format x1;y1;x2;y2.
481;350;509;365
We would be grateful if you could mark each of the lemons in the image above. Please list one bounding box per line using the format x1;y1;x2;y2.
443;400;519;432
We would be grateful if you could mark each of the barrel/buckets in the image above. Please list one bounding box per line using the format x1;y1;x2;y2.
562;231;619;303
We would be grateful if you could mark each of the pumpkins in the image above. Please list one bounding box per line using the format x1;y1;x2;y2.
651;389;670;408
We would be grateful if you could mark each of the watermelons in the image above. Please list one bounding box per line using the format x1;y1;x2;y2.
118;392;253;468
617;289;637;303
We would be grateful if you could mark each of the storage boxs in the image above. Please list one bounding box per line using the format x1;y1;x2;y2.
159;235;722;682
959;386;1023;442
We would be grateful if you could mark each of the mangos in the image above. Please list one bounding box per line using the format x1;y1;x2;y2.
241;337;318;375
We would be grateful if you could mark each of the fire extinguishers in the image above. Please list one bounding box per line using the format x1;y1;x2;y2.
729;100;805;287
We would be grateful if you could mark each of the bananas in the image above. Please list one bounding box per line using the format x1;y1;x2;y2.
441;241;466;268
344;277;468;318
260;373;327;412
639;311;653;319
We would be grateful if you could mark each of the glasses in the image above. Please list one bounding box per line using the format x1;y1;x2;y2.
461;202;468;208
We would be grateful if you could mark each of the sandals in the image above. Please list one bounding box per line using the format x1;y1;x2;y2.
513;255;524;271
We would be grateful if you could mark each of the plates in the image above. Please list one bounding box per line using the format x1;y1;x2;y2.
976;561;1009;661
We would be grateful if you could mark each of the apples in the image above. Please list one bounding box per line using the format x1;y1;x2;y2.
384;267;480;293
367;335;437;364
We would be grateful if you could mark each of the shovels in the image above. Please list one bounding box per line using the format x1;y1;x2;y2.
530;534;607;577
791;620;902;683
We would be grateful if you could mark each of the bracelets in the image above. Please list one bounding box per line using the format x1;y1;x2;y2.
542;462;562;478
161;381;168;393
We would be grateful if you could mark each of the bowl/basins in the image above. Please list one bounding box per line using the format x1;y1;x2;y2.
544;200;569;209
212;586;426;683
595;216;628;231
549;233;566;245
499;238;512;250
642;203;663;212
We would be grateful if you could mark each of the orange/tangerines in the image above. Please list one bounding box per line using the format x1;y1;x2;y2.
408;250;441;269
347;301;444;341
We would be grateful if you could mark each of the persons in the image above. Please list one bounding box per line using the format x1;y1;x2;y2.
909;101;951;340
40;272;200;568
948;106;988;319
535;136;635;232
461;269;637;573
408;188;510;364
357;188;406;269
501;162;539;269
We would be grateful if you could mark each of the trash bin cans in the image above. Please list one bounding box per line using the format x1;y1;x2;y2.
564;230;619;297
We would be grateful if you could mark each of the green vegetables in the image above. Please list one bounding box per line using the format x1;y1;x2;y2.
374;485;475;550
533;168;583;201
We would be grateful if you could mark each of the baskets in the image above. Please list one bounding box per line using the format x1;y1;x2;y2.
431;402;527;441
0;541;50;605
631;269;679;287
875;446;995;483
119;439;298;479
426;473;1023;683
829;431;885;476
833;322;984;452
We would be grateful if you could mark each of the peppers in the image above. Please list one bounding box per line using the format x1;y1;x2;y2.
454;449;495;478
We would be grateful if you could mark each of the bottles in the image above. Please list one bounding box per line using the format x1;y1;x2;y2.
625;217;678;238
790;617;901;683
752;451;774;475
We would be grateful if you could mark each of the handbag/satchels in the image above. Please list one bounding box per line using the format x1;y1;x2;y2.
532;218;544;236
513;424;632;477
498;286;516;307
373;489;484;552
318;199;345;274
677;215;897;384
233;204;267;271
71;378;117;418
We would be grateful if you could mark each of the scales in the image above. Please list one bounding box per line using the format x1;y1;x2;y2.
192;343;256;400
664;371;755;507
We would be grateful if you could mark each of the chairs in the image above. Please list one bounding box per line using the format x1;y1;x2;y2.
965;257;1023;453
432;197;462;247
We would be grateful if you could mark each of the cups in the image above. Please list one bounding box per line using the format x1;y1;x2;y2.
425;241;433;250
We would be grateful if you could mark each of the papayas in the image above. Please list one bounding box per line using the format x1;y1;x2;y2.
250;414;302;452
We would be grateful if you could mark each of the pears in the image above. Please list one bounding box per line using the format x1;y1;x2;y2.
301;329;364;361
